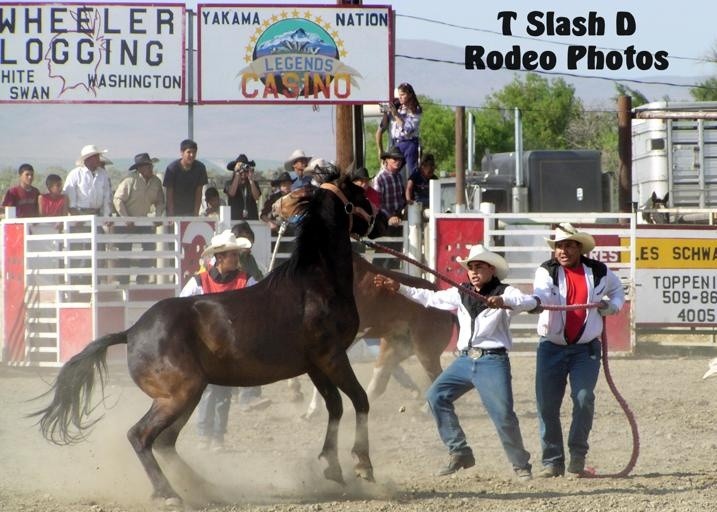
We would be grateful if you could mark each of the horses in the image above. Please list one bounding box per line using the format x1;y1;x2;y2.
271;186;462;421
637;191;669;224
21;158;391;512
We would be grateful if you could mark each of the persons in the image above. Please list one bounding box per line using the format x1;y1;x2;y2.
303;146;438;271
112;150;165;287
285;147;317;225
231;223;271;414
529;220;628;479
370;244;539;486
200;181;227;216
60;146;111;302
223;152;265;223
374;97;409;188
176;229;252;452
382;82;422;177
362;334;421;401
259;170;298;227
163;139;207;284
35;175;71;218
1;163;38;217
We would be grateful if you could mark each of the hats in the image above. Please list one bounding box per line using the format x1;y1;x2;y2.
227;154;255;170
129;153;157;170
380;147;404;160
201;234;252;256
272;150;328;186
75;145;107;164
544;223;595;255
456;244;508;281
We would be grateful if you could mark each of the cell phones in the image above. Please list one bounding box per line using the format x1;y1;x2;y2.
379;102;391;112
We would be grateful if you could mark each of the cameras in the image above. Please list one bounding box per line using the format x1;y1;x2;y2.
241;162;252;173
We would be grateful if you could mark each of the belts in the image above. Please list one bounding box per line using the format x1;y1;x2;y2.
462;349;503;355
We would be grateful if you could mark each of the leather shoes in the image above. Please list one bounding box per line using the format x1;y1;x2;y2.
440;452;475;474
516;462;584;481
